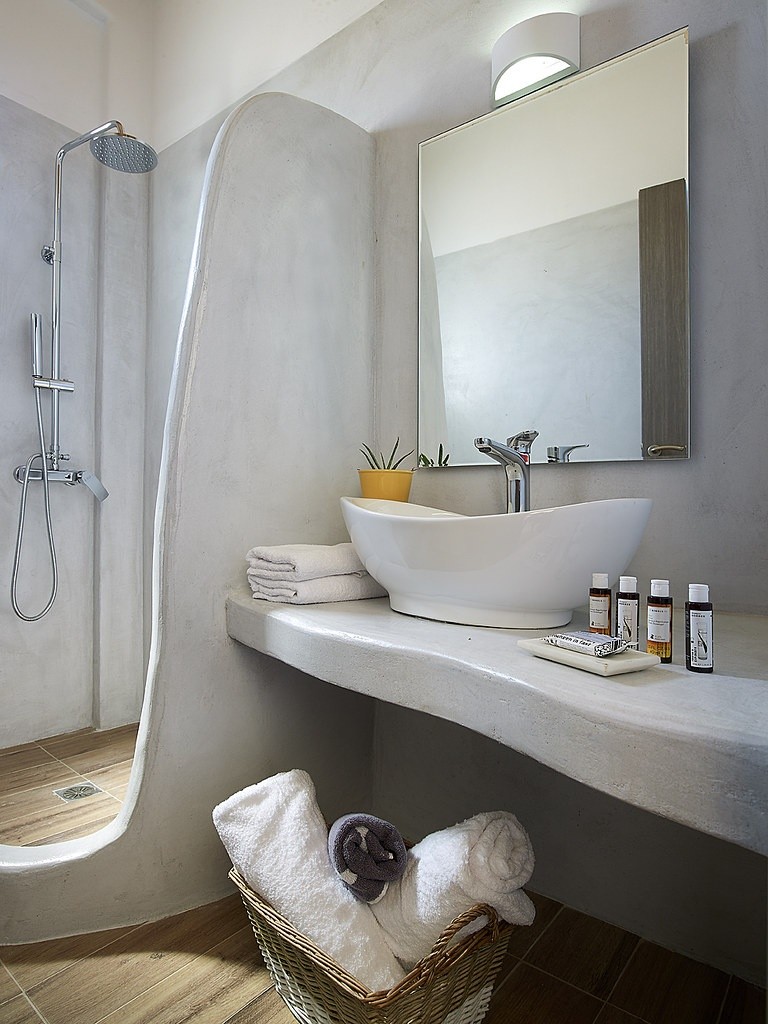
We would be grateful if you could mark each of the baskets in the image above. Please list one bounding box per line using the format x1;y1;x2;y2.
228;837;514;1023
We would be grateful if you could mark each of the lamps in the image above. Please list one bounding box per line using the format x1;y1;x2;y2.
488;12;582;110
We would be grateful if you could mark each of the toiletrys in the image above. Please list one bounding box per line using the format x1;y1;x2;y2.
585;572;717;673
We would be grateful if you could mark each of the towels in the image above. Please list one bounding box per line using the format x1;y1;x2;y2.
326;813;406;903
247;573;390;602
246;542;372;581
212;767;406;997
373;809;539;971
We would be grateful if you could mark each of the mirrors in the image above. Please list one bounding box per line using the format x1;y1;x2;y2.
413;25;693;448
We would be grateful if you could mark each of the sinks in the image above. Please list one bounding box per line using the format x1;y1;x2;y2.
340;495;654;631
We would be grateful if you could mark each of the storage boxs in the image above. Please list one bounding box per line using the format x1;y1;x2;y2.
228;866;515;1024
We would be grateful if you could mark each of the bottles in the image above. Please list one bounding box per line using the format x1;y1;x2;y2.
615;575;640;650
646;578;674;664
590;572;611;636
683;583;715;673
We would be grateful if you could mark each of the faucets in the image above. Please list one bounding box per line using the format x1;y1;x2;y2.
473;430;541;517
545;442;587;462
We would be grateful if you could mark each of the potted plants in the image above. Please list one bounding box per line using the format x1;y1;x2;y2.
357;437;415;502
418;443;450;468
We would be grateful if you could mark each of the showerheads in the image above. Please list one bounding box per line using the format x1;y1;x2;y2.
30;312;47;381
58;119;162;173
